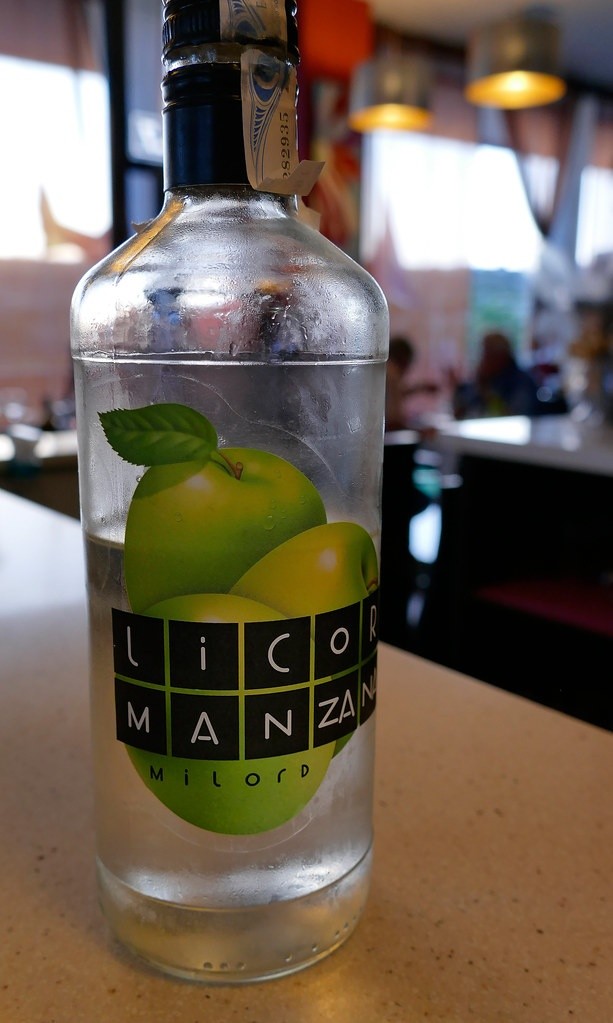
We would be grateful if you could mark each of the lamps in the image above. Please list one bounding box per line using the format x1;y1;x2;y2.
465;19;567;110
349;45;435;132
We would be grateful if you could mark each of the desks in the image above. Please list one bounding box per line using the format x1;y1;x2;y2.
0;487;613;1023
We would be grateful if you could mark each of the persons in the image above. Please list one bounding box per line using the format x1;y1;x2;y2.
383;300;613;594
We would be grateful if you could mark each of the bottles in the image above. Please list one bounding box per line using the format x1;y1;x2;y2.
68;0;391;986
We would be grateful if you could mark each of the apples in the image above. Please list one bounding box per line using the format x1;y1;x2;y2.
118;445;379;835
